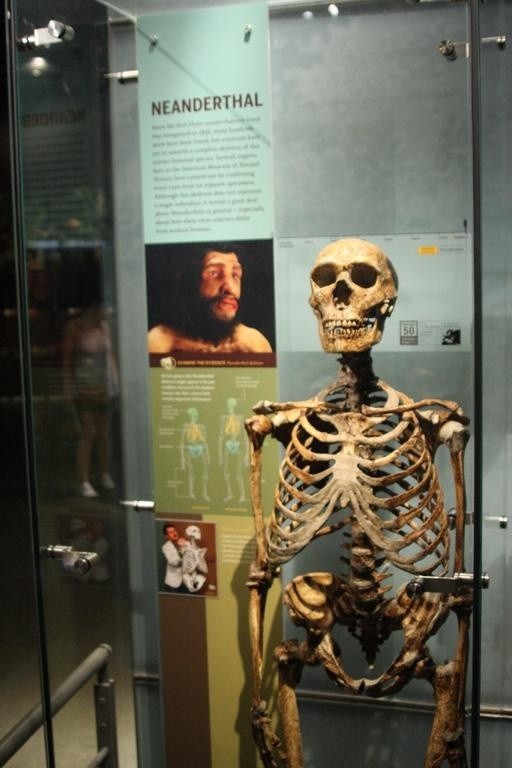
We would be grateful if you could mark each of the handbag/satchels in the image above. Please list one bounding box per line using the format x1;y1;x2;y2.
77;384;110;404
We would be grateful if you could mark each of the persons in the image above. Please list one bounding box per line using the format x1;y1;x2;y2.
148;242;272;354
82;522;110;583
62;289;120;498
161;524;186;593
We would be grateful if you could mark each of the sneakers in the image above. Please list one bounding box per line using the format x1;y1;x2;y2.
101;474;115;490
79;481;98;498
